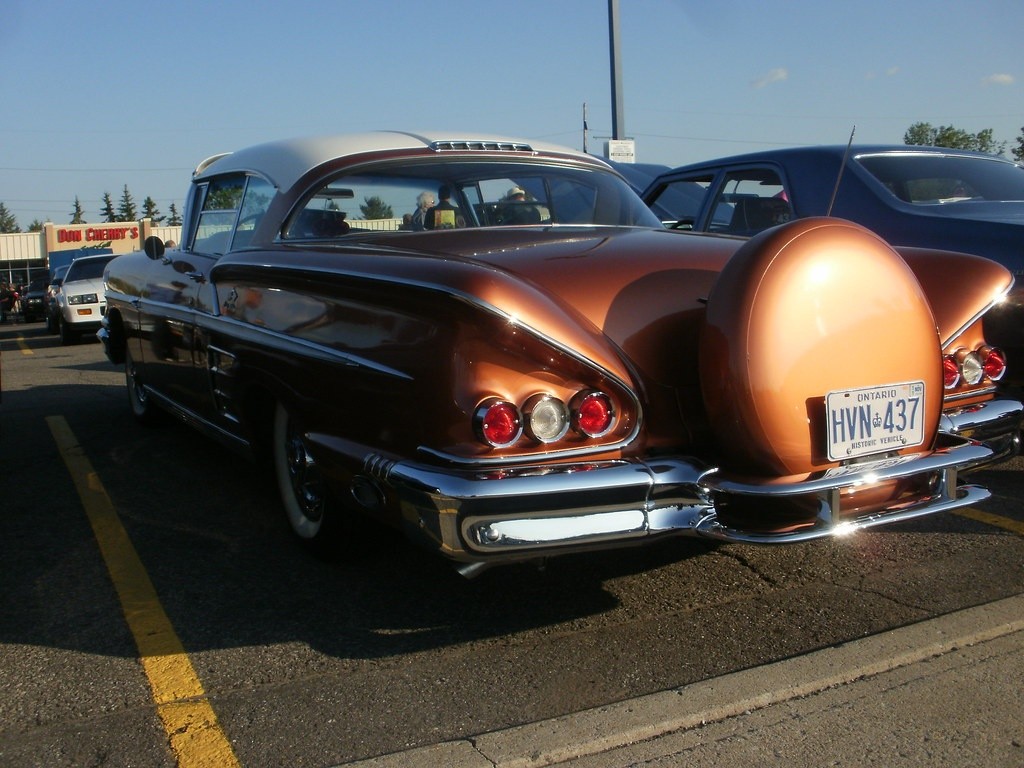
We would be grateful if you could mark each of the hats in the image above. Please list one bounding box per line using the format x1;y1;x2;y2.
506;186;526;199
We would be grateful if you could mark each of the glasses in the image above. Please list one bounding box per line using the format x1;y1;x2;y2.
428;200;435;205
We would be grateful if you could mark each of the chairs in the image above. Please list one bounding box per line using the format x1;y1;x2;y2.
285;197;793;236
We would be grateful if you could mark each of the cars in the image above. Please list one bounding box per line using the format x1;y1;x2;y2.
46;253;124;343
43;264;71;335
96;129;1024;594
585;148;1024;416
20;277;49;324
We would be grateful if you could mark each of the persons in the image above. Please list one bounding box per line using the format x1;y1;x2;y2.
165;240;176;248
500;187;541;225
398;214;413;230
424;185;462;230
411;191;435;232
0;279;24;313
